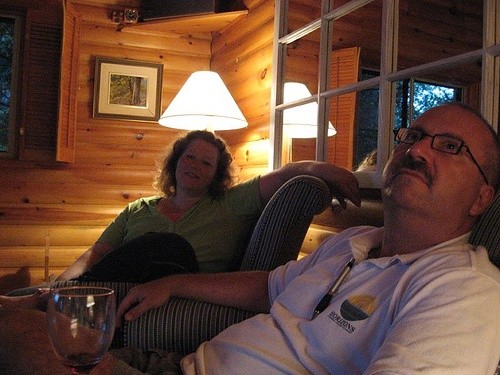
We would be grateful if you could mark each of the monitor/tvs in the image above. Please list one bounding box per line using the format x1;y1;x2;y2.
141;0;220;22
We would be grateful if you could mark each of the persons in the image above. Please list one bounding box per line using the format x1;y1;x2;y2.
0;129;362;320
0;102;500;375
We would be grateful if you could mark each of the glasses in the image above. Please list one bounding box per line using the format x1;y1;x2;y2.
392;128;491;186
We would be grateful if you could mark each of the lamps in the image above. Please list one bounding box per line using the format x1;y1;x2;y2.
157;70;249;134
283;82;338;139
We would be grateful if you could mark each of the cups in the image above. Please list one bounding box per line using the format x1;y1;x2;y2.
46;285;116;375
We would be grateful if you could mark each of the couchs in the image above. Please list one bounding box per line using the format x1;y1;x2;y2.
51;175;333;356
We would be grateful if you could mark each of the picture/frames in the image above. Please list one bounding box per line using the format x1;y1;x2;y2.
92;54;165;124
